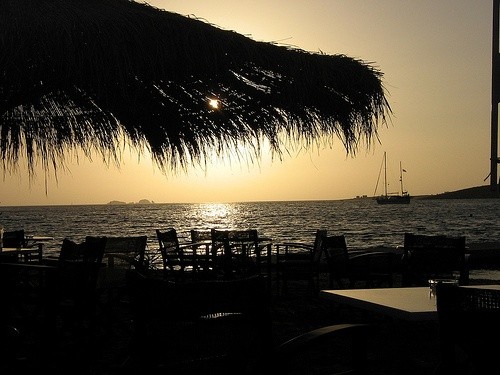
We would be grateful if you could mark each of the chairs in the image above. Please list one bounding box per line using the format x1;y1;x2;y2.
0;230;148;299
155;226;273;308
275;228;472;284
273;320;374;362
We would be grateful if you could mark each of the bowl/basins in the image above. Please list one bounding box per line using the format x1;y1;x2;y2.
428;279;457;289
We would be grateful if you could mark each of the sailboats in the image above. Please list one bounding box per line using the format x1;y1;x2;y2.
371;152;416;205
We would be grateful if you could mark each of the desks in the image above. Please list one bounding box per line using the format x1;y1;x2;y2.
457;275;500;299
314;280;439;317
